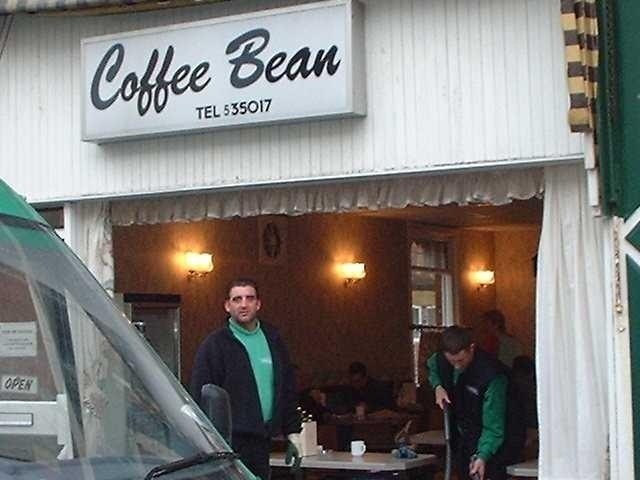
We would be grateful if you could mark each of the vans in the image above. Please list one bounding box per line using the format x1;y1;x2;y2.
1;177;260;480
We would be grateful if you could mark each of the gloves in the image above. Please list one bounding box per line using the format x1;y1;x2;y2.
285;433;303;474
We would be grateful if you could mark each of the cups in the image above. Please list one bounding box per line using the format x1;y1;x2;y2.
351;440;366;457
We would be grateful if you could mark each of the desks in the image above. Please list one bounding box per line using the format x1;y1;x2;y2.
270;414;538;479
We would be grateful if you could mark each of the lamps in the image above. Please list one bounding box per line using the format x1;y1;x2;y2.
344;263;365;288
476;270;494;291
186;252;212;279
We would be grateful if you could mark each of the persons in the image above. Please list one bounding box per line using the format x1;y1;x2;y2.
498;353;538;467
483;308;529;367
426;325;516;479
339;361;384;421
288;362;335;422
183;277;305;480
